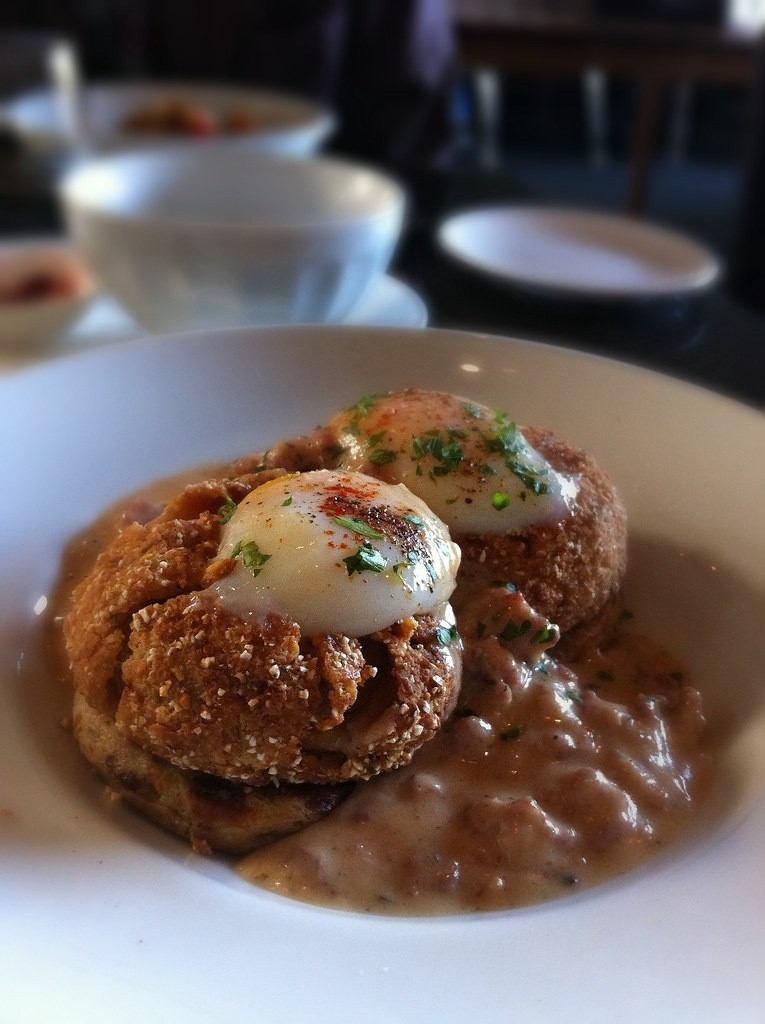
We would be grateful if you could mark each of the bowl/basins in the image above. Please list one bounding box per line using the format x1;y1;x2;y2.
7;80;337;184
56;150;403;324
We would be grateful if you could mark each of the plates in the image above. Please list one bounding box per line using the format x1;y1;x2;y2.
437;206;719;298
46;273;443;340
0;325;765;1024
